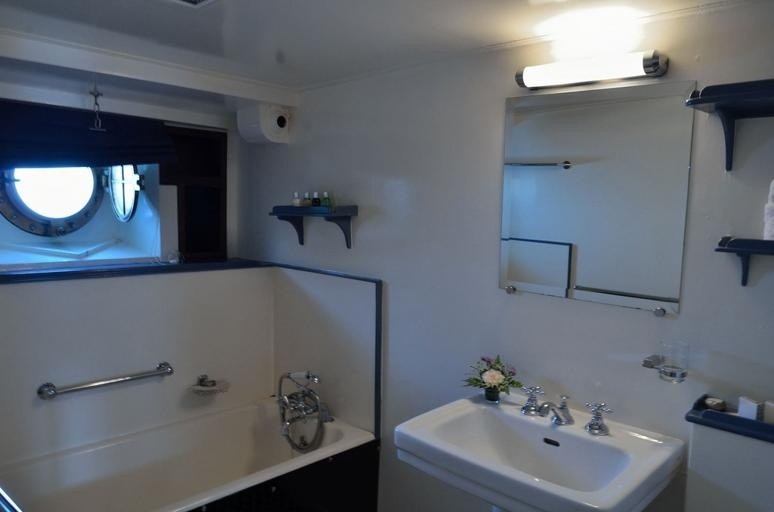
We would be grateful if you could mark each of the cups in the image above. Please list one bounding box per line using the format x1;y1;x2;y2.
661;334;689;385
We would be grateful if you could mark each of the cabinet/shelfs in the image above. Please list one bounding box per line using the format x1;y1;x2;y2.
684;76;770;286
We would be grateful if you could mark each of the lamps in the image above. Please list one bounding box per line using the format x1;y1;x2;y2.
515;47;668;91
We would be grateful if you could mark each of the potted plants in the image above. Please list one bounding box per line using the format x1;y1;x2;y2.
459;354;523;402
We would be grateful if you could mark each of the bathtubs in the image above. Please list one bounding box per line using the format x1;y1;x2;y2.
0;396;375;512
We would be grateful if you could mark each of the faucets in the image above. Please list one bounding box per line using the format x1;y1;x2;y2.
281;414;305;437
538;394;575;425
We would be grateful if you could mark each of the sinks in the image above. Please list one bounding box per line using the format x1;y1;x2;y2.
395;390;685;512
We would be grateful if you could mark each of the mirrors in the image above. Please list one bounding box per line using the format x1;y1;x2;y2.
492;79;699;316
